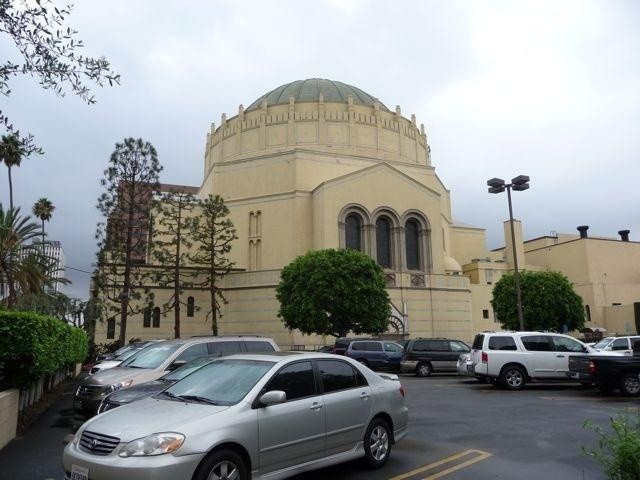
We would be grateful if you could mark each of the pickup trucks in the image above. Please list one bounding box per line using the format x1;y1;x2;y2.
565;339;640;397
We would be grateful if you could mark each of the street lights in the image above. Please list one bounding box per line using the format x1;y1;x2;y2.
488;176;529;330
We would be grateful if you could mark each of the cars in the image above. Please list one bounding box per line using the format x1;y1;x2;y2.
61;334;281;416
318;337;475;376
584;335;640;357
62;350;410;479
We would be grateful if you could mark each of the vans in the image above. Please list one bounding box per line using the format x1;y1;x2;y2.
469;330;600;391
